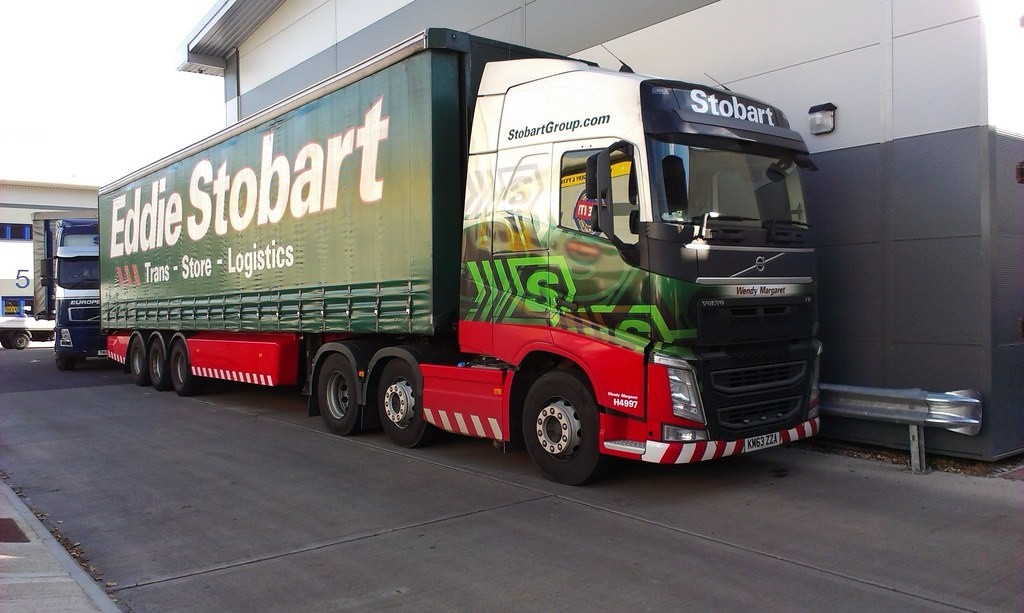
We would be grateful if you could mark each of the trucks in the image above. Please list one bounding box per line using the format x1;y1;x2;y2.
0;295;56;350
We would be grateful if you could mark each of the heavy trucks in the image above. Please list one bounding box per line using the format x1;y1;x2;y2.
98;28;825;488
40;218;108;371
